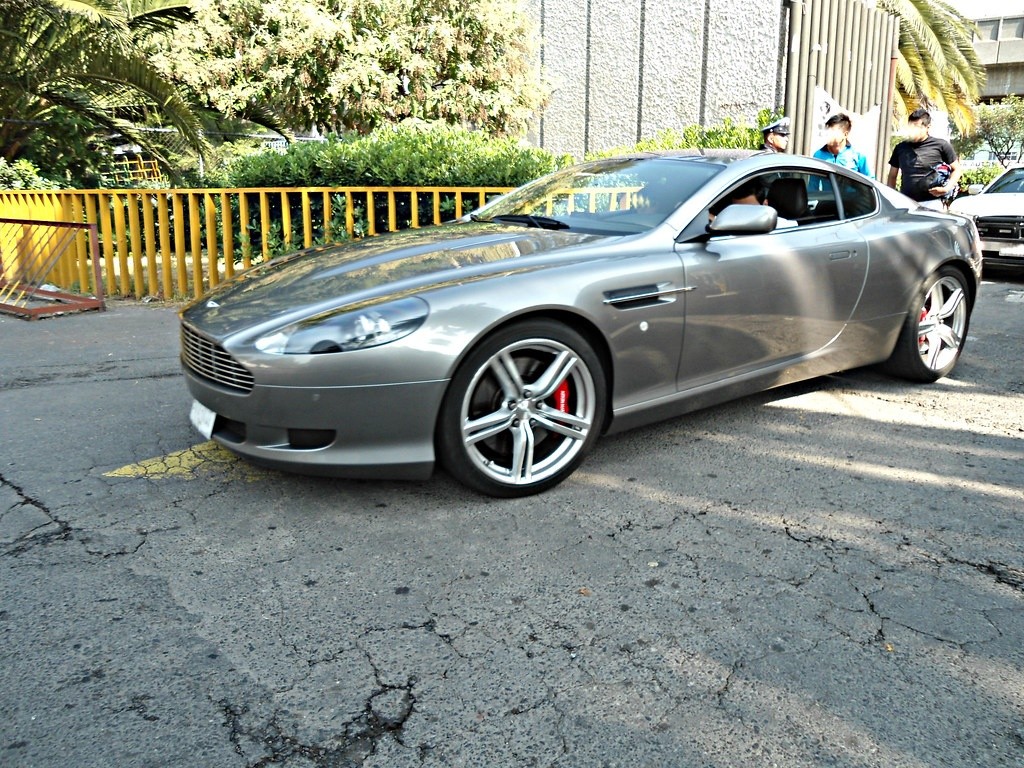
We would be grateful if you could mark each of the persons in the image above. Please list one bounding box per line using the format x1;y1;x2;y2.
887;109;963;210
755;116;795;198
805;113;875;195
707;175;799;233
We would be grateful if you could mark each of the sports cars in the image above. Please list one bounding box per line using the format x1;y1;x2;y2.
177;149;985;500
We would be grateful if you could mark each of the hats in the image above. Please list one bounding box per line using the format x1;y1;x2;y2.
761;117;791;135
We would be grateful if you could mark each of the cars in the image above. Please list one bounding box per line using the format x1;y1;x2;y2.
946;162;1024;278
